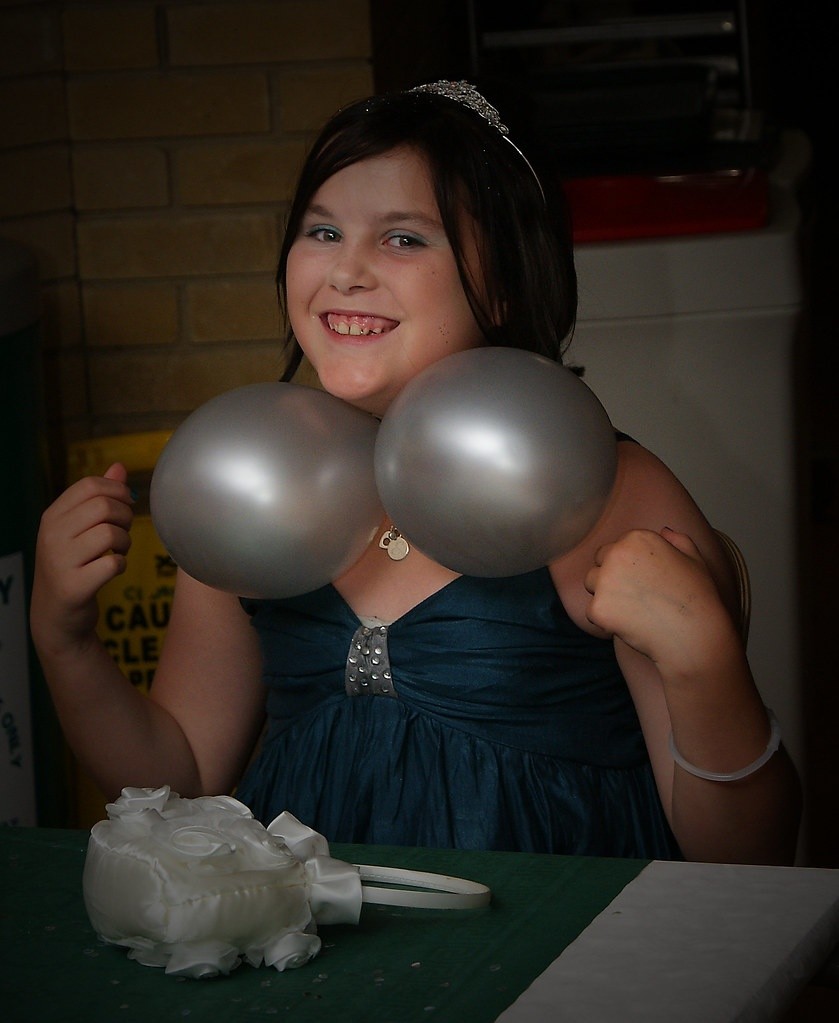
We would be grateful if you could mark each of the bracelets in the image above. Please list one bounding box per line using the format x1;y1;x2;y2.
665;702;782;780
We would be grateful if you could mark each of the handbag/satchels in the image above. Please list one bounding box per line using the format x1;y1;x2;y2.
83;787;488;978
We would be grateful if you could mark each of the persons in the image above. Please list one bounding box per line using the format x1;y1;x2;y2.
28;70;798;869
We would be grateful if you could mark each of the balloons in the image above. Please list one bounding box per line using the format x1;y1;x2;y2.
375;345;618;577
149;377;379;598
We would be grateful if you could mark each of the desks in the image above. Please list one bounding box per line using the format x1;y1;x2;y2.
0;820;839;1023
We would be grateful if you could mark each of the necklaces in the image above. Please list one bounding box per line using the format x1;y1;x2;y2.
378;529;410;560
